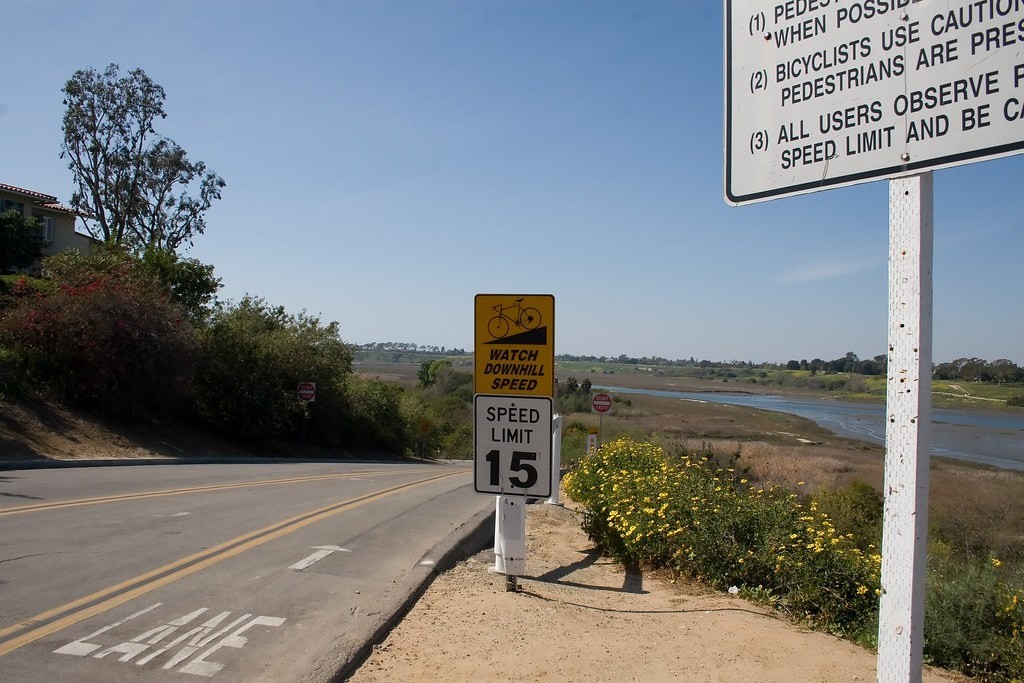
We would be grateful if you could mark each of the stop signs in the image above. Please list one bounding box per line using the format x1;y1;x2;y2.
298;382;315;403
592;391;612;414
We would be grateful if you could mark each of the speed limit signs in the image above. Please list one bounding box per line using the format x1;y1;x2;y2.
471;393;553;501
